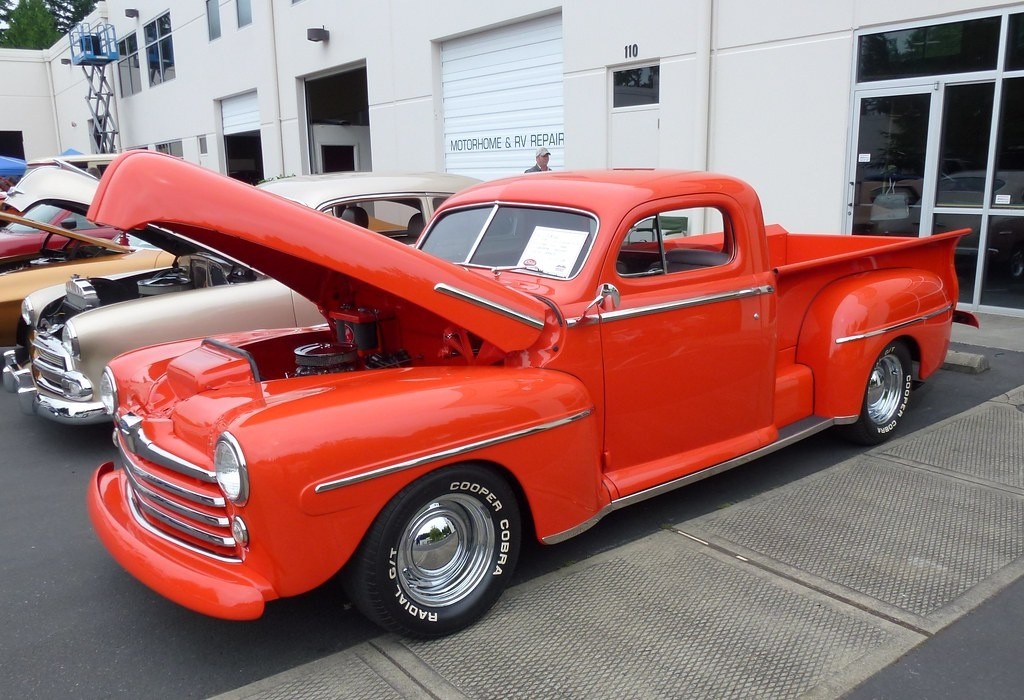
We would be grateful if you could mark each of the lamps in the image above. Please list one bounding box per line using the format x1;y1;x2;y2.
307;25;329;42
61;59;71;64
125;9;139;17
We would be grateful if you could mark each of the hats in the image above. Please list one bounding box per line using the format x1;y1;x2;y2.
536;147;551;158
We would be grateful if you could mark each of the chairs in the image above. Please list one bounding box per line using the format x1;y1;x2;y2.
628;216;688;244
648;247;732;276
395;213;425;245
340;206;370;232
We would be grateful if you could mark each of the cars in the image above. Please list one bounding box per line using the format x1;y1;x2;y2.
2;134;1021;642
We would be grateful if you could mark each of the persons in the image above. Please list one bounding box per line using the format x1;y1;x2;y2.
523;146;553;173
